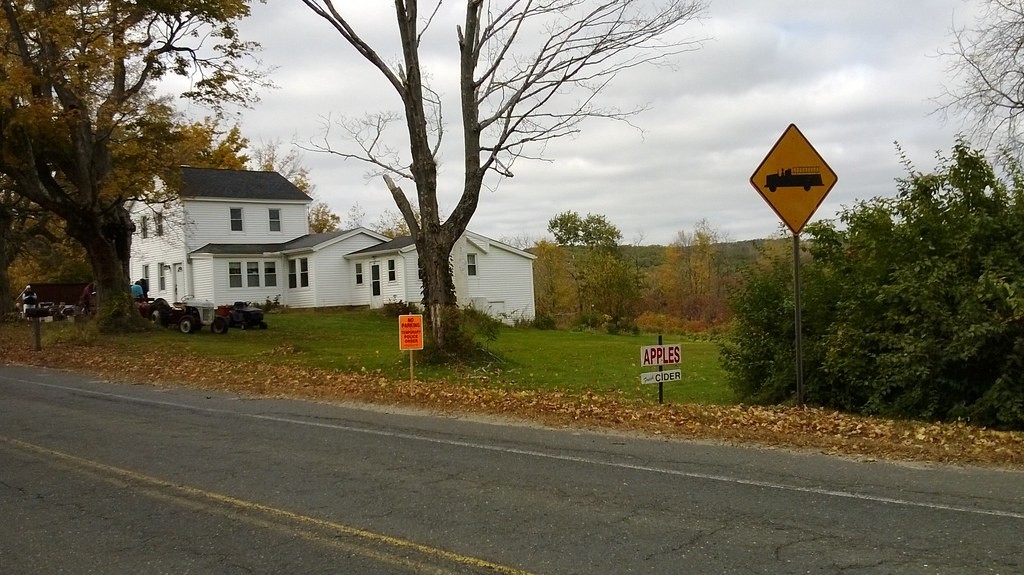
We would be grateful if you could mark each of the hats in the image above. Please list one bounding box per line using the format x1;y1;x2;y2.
26;285;31;289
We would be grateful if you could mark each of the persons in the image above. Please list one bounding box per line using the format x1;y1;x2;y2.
22;285;37;322
131;279;149;302
